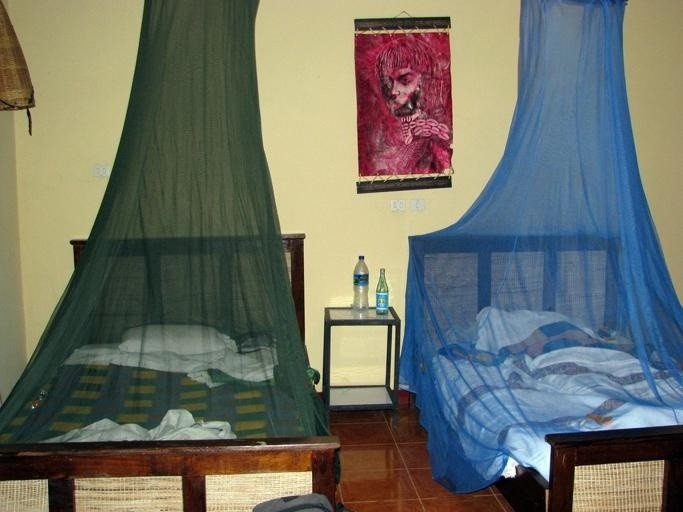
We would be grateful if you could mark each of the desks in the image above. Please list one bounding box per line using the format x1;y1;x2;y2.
322;307;402;431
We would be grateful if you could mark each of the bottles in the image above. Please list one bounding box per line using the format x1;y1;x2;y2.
375;268;389;316
353;255;369;314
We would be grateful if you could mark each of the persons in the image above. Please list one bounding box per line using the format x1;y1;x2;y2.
366;37;452;177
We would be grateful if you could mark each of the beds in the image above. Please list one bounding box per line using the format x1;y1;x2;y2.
406;232;683;511
3;233;344;512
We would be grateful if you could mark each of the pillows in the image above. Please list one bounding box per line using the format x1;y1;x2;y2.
474;305;597;354
117;323;227;356
